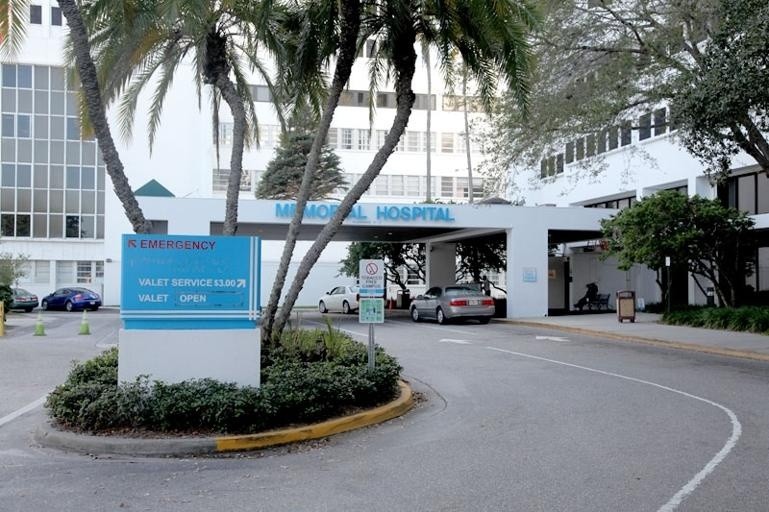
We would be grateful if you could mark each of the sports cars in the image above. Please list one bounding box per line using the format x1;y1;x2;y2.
41;287;102;312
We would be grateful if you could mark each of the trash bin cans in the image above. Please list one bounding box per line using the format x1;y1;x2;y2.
616;289;635;323
396;289;410;309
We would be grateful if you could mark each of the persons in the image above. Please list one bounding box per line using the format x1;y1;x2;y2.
469;276;479;289
573;281;597;311
480;275;491;298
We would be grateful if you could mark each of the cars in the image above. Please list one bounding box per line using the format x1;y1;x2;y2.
409;285;495;324
9;288;39;313
318;284;387;314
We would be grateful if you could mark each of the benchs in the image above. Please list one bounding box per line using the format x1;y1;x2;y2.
590;294;611;310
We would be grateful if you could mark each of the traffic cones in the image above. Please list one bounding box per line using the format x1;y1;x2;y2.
78;309;92;335
388;296;395;310
32;311;47;336
